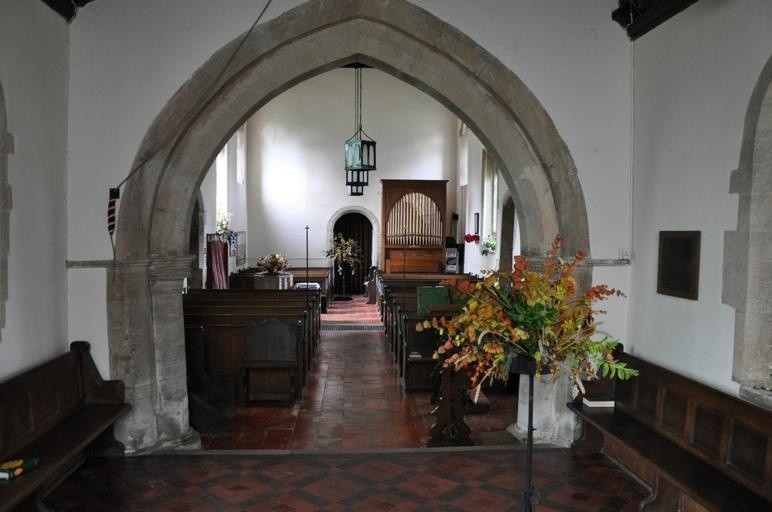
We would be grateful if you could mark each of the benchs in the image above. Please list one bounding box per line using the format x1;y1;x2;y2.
363;266;382;304
0;333;131;512
374;270;488;395
183;264;333;411
565;343;772;512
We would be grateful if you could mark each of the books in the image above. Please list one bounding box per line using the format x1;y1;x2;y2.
582;393;616;408
0;456;41;482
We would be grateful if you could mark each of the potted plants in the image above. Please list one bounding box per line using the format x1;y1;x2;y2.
414;233;640;443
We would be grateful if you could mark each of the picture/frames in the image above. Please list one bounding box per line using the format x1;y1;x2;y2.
236;231;247;267
657;228;702;302
474;213;479;234
229;239;236;257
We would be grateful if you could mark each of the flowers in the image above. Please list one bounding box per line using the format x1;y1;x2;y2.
463;233;480;243
256;251;289;271
214;207;239;244
478;234;497;257
321;231;367;279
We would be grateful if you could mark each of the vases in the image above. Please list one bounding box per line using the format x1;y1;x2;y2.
341;261;348;299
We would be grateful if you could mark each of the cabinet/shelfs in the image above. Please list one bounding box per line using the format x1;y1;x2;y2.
377;178;447;274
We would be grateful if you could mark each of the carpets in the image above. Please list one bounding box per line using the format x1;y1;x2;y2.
32;444;656;511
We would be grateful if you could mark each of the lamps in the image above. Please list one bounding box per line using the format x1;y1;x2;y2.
343;60;375;198
